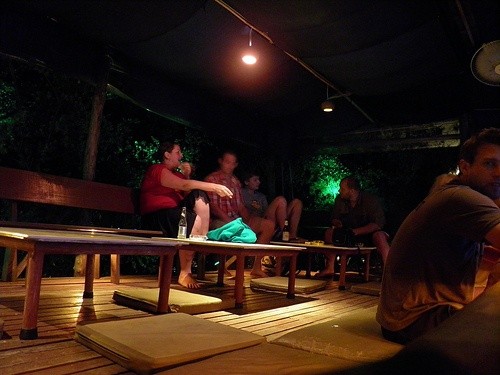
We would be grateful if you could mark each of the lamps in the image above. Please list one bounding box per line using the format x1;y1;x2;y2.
321;85;335;112
241;29;259;66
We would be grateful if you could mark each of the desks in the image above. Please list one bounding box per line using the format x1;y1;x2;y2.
0;227;191;341
150;235;305;309
270;240;377;290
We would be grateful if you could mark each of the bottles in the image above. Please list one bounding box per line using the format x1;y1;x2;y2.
177;207;187;239
282;218;290;242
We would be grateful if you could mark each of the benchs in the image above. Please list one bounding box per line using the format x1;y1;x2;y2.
0;167;386;284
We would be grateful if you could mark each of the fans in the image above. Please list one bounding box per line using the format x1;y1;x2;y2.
470;40;500;86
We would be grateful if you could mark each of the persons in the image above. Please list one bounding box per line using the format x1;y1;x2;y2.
139;140;234;288
204;150;274;277
314;175;392;279
376;128;500;375
242;173;308;243
